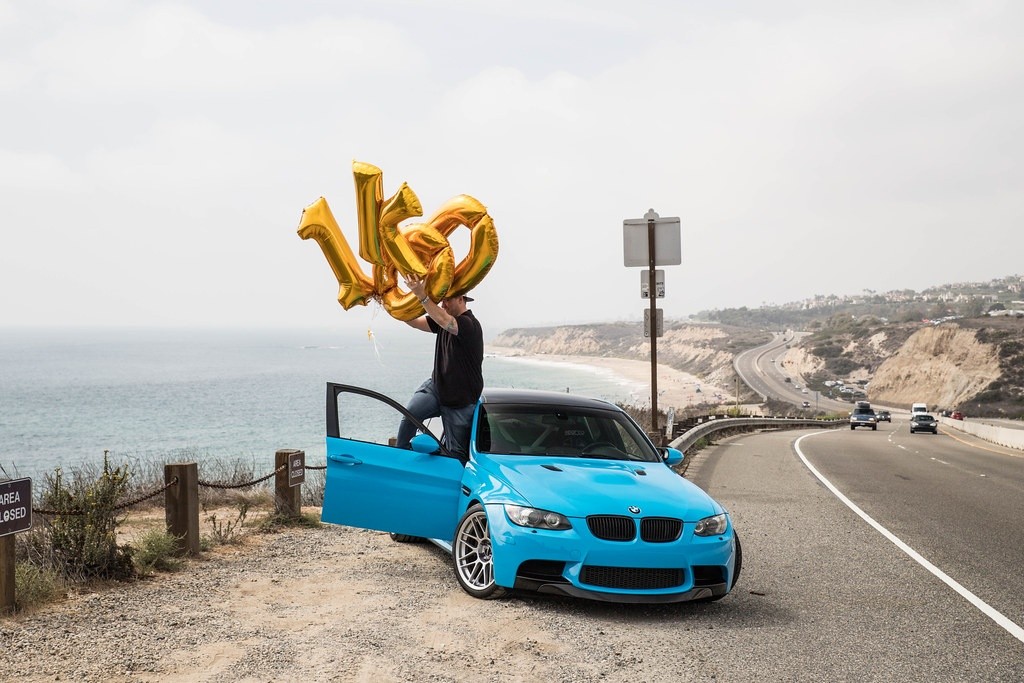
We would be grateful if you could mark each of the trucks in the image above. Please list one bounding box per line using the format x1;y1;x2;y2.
854;400;870;408
910;403;929;421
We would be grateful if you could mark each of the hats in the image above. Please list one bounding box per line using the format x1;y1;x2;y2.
463;292;474;301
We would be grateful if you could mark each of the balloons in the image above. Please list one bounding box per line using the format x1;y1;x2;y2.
296;158;499;320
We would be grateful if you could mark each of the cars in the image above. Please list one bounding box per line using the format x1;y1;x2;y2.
875;410;892;423
321;382;743;606
909;414;939;434
848;407;879;431
941;410;964;420
769;329;812;409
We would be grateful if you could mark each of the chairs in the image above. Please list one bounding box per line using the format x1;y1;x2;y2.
556;421;592;451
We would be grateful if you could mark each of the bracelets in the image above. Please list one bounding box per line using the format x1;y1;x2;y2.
419;296;429;303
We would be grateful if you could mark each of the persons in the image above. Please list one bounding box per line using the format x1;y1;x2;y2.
395;273;484;460
